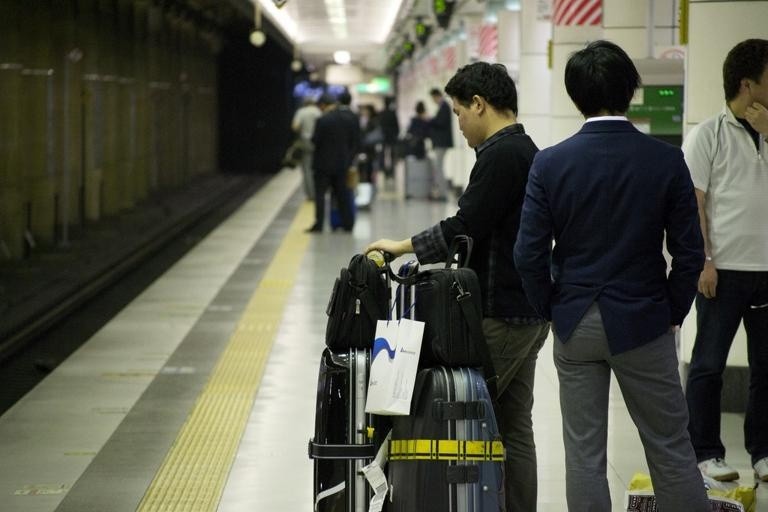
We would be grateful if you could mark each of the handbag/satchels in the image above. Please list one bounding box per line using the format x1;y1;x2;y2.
397;235;484;367
327;254;391;352
627;474;756;512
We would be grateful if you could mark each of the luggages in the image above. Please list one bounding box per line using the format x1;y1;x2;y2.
309;250;392;512
386;260;507;512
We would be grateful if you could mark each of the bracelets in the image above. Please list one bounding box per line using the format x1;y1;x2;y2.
702;255;711;264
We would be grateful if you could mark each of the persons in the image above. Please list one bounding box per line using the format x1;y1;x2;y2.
290;85;453;234
512;38;704;511
363;62;543;511
679;39;768;488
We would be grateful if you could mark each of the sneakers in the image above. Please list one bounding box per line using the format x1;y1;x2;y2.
698;459;740;481
